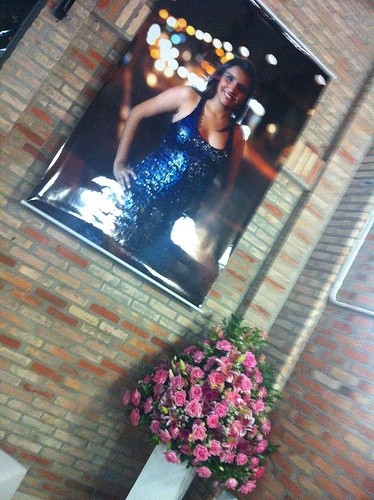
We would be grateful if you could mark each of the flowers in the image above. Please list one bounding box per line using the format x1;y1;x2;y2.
121;313;283;495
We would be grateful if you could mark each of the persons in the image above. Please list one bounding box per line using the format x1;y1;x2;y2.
108;56;255;258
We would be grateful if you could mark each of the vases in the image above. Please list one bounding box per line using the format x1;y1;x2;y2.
124;442;197;500
